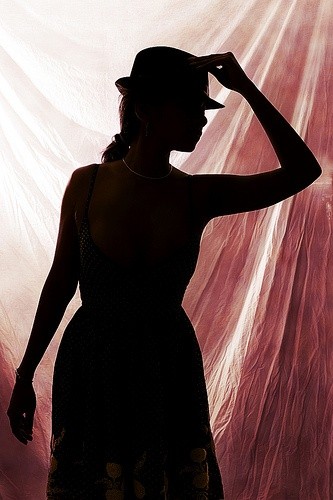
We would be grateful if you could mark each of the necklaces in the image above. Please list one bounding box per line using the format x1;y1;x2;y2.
120;155;167;179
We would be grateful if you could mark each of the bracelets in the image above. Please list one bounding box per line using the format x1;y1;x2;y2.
16;369;32;382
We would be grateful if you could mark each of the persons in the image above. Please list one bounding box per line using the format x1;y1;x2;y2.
6;45;323;500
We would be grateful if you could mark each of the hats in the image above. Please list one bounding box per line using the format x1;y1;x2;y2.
114;47;224;110
